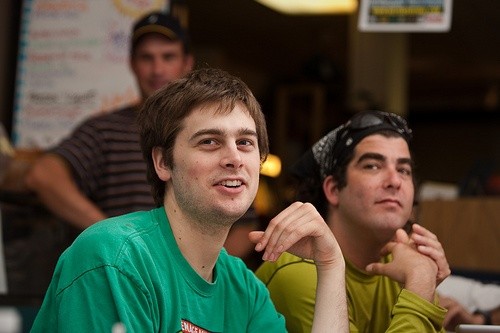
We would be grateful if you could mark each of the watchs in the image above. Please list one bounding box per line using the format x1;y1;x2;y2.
468;306;491;325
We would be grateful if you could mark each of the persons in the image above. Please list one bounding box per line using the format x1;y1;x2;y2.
255;111;451;333
439;297;500;328
30;66;349;333
24;12;259;257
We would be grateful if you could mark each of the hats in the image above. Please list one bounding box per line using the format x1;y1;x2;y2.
311;111;412;181
132;10;181;42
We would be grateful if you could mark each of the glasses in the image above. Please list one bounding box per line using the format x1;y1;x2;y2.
328;110;414;170
